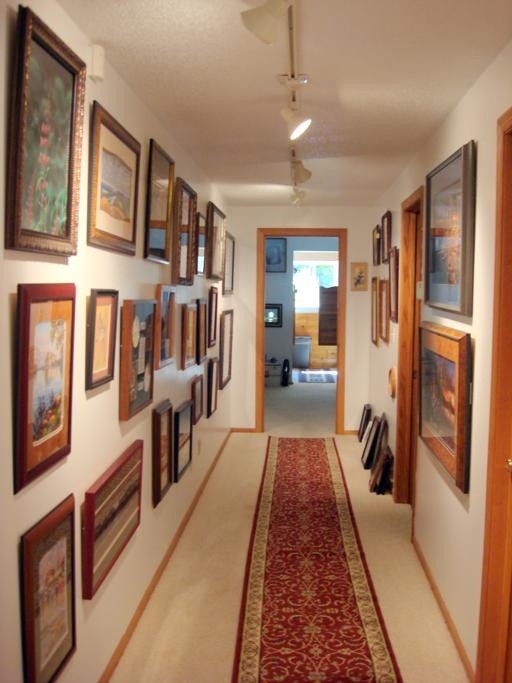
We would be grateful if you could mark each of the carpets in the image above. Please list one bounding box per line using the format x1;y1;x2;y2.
298;373;335;383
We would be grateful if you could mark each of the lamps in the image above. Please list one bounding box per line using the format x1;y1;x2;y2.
236;3;316;211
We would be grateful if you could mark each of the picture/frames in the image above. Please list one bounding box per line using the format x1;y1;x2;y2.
264;303;282;327
265;237;287;272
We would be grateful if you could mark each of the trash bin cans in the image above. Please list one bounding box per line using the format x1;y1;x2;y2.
293;337;312;368
